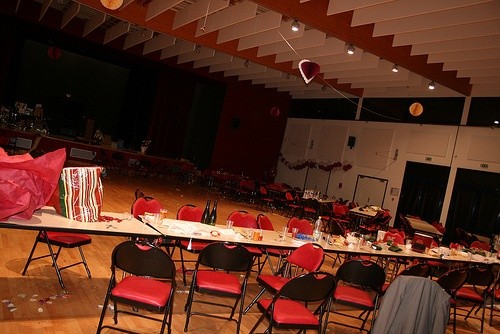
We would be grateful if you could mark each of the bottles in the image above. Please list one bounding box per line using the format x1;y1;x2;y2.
201;200;210;224
207;200;218;226
312;216;323;241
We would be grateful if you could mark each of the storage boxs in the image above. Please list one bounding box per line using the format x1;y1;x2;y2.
411;233;432;253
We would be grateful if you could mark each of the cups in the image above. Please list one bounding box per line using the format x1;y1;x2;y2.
159;209;167;220
253;230;260;241
292;227;299;238
281;226;289;241
227;220;233;229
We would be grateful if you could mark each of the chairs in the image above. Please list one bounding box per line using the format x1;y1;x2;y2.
22;180;500;334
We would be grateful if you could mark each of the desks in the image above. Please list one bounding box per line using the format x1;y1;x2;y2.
278;230;442;286
267;186;291;212
349;206;380;225
431;246;500;295
317;199;337;208
138;215;307;315
0;206;162;312
468;233;490;244
406;217;443;238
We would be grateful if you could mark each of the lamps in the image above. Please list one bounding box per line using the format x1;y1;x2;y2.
392;64;399;72
292;20;300;32
428;81;435;90
347;44;355;55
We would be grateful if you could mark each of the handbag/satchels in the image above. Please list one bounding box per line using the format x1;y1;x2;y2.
59;167;102;222
